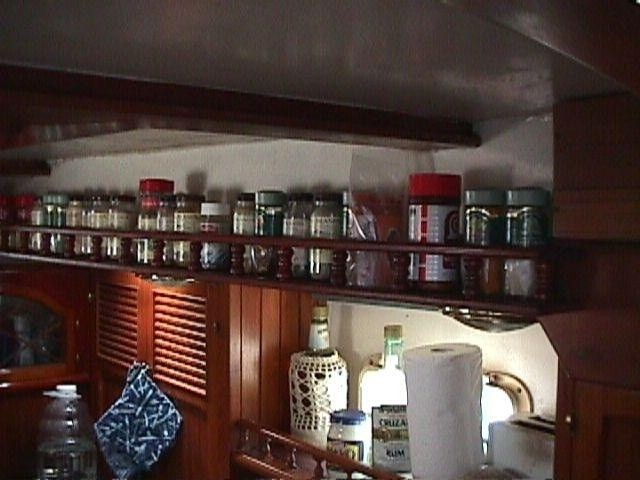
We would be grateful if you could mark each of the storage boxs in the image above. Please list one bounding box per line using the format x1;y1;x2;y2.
552;95;640;242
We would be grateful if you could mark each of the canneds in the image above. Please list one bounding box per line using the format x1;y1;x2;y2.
0;191;341;281
462;187;554;298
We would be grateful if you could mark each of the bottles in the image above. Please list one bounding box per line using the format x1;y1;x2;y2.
325;407;371;480
288;299;348;451
357;324;413;472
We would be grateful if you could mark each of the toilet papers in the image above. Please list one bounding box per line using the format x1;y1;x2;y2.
399;342;486;479
